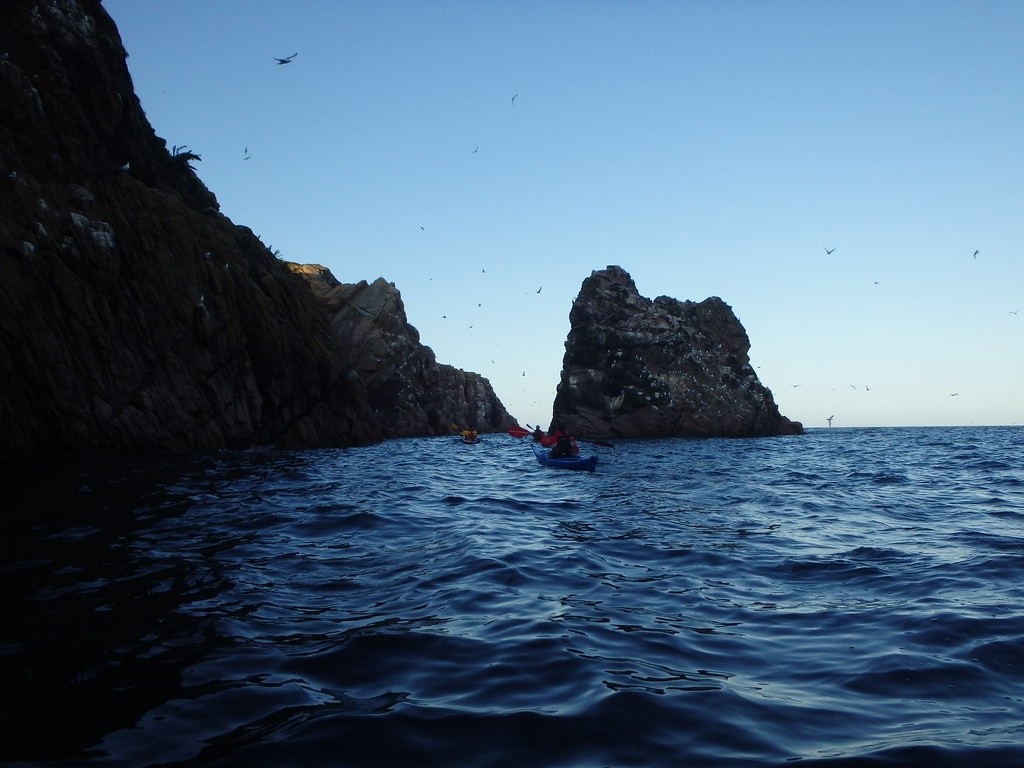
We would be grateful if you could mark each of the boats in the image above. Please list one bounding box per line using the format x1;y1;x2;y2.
529;443;599;472
461;434;480;444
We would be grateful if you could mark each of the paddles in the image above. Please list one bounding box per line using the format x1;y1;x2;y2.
547;432;614;448
526;424;536;432
452;424;463;433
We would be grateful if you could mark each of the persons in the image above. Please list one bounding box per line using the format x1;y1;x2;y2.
540;422;579;458
472;429;478;438
532;425;544;442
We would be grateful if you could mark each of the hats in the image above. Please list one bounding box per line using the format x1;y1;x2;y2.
536;425;540;429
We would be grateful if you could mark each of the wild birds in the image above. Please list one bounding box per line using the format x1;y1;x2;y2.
473;146;478;153
273;53;297;66
826;415;834;427
512;94;518;105
243;147;251;160
420;226;542;376
792;249;1020;396
823;247;836;256
